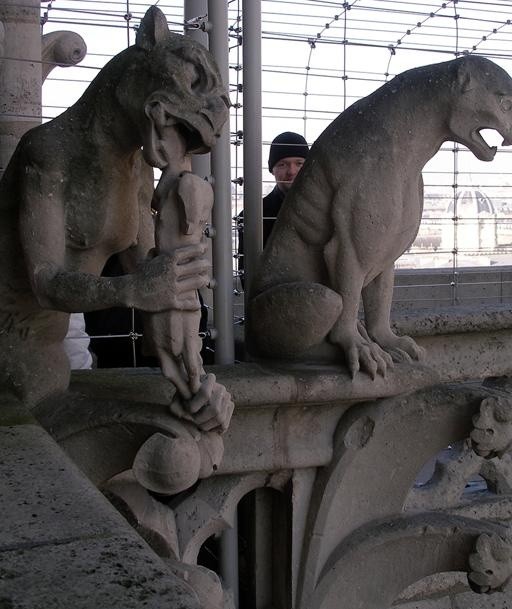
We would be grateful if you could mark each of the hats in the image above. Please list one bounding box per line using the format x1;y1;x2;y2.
267;132;310;171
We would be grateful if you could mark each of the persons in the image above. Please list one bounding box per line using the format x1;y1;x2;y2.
83;251;209;371
231;131;317;293
